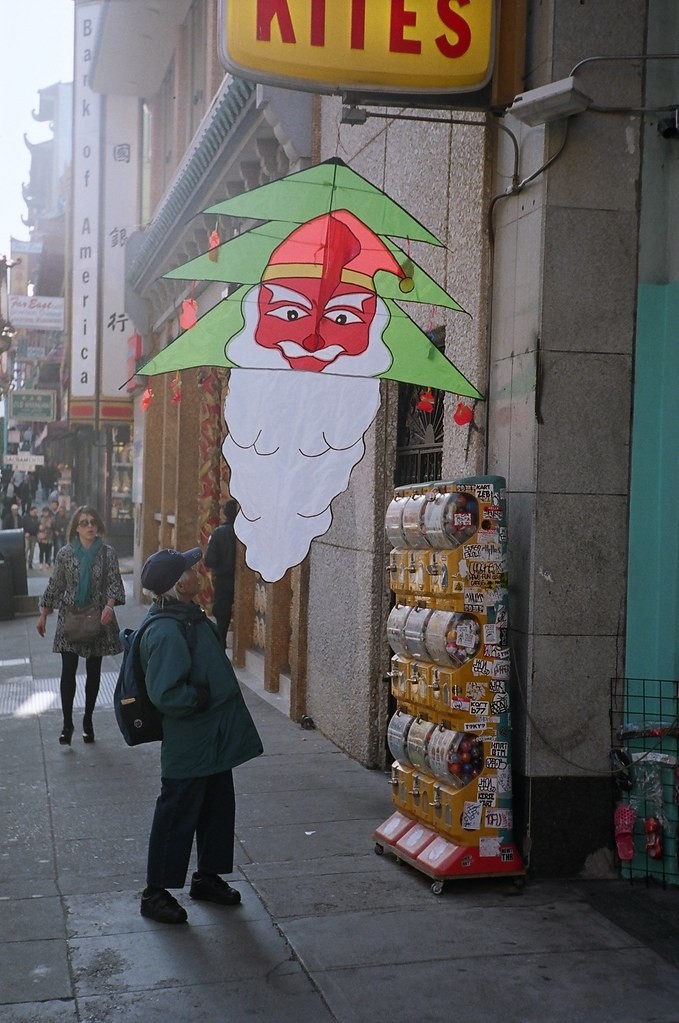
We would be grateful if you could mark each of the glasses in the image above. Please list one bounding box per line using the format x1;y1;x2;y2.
76;519;98;528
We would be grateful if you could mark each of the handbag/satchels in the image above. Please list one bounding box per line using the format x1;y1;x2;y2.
63;603;102;644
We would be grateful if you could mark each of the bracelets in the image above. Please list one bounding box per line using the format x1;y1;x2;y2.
105;604;113;612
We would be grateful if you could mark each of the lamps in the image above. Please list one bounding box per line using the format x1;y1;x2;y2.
506;76;594;127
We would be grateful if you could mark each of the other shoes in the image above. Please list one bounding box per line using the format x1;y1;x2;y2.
46;563;50;569
39;563;42;569
29;564;34;569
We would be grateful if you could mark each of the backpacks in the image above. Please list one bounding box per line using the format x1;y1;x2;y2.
115;609;197;746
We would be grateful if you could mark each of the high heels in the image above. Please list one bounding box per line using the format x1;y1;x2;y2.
59;723;74;746
82;716;95;744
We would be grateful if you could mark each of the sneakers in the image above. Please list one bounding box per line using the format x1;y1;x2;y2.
141;883;189;925
189;870;241;905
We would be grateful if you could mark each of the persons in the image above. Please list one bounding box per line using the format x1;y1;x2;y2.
140;547;264;923
36;506;126;747
0;464;61;516
3;501;68;569
204;500;241;650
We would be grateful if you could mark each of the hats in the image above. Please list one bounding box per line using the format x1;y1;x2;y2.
224;500;238;518
139;545;203;593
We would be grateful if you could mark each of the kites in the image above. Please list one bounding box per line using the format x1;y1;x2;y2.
121;156;487;583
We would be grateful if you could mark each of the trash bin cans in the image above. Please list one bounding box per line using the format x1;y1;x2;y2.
0;528;29;596
